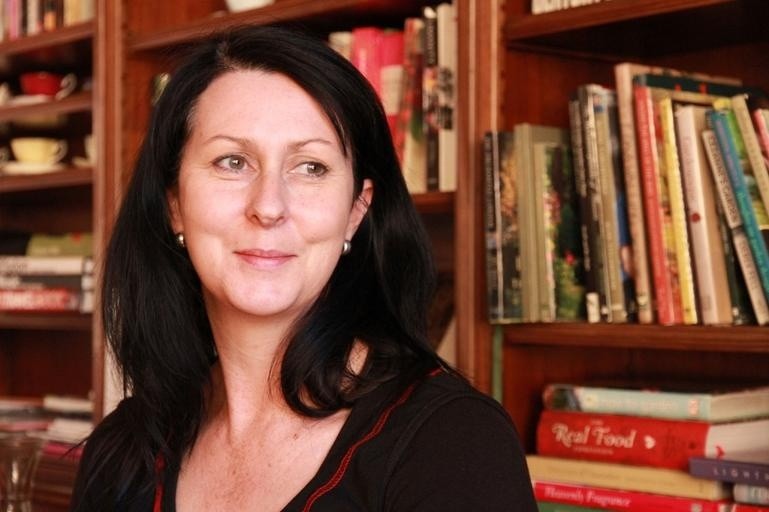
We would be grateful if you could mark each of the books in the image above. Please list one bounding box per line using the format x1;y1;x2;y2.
1;234;95;314
327;3;456;194
483;64;769;325
0;0;95;39
525;371;769;512
1;396;93;458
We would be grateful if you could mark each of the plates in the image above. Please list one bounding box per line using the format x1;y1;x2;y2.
3;161;63;176
72;156;94;169
7;94;50;107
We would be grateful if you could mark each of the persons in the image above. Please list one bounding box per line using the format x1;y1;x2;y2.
67;26;540;512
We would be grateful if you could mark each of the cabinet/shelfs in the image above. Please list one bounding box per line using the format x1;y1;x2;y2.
0;0;769;512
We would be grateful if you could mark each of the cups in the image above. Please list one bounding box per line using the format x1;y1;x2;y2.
9;136;66;165
83;134;92;158
17;71;78;99
0;438;46;511
